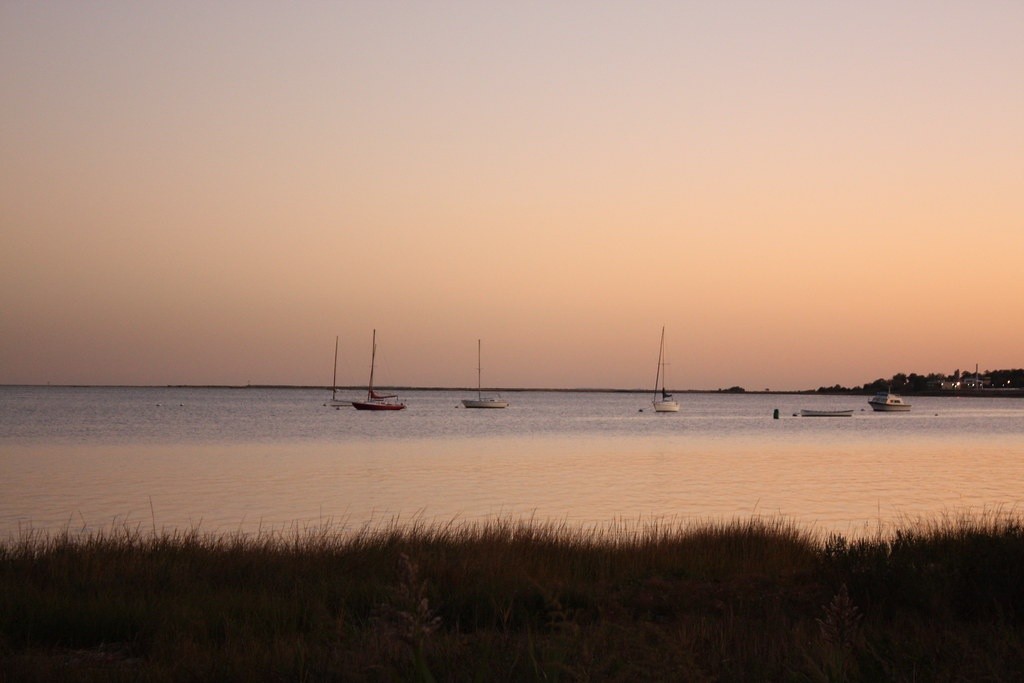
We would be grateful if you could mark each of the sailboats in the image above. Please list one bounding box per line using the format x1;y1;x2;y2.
650;327;680;412
350;328;407;411
323;335;352;409
460;339;510;410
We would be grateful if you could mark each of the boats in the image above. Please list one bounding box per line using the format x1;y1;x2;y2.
799;407;854;417
868;379;912;412
